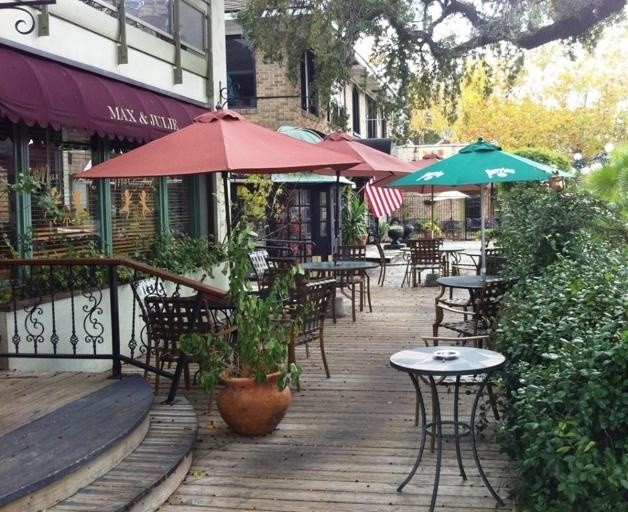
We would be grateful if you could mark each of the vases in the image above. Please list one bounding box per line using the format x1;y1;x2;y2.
355;234;368;245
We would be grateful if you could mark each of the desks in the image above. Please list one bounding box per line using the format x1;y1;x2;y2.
435;276;503;338
390;347;506;512
400;248;465;288
299;262;380;311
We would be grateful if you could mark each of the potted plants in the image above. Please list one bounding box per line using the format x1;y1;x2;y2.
178;215;316;436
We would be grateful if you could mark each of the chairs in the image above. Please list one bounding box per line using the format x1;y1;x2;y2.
131;277;206;389
265;257;337;324
330;269;355;322
415;335;501;426
326;245;372;313
267;279;336;392
247;250;297;318
408;239;449;286
452;247;504;276
435;276;520;348
146;297;226;396
452;239;490;276
